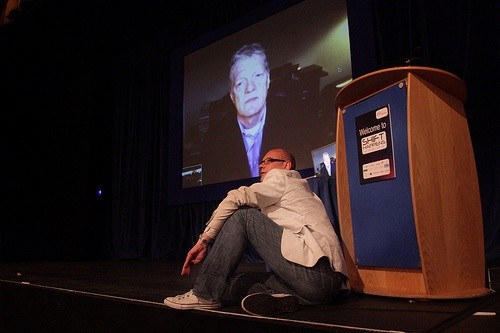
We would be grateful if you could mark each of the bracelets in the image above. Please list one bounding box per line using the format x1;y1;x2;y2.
199;234;210;245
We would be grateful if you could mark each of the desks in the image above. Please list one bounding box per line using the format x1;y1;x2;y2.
304;174;339;236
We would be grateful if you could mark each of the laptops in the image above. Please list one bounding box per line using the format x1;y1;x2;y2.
303;142;336;180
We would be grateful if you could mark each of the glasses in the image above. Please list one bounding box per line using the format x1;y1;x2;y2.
259;157;286;165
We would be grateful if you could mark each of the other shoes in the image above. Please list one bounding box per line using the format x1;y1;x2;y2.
241;290;299;316
164;289;219;311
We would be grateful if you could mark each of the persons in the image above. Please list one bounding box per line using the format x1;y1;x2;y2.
202;43;329;183
163;148;350;311
